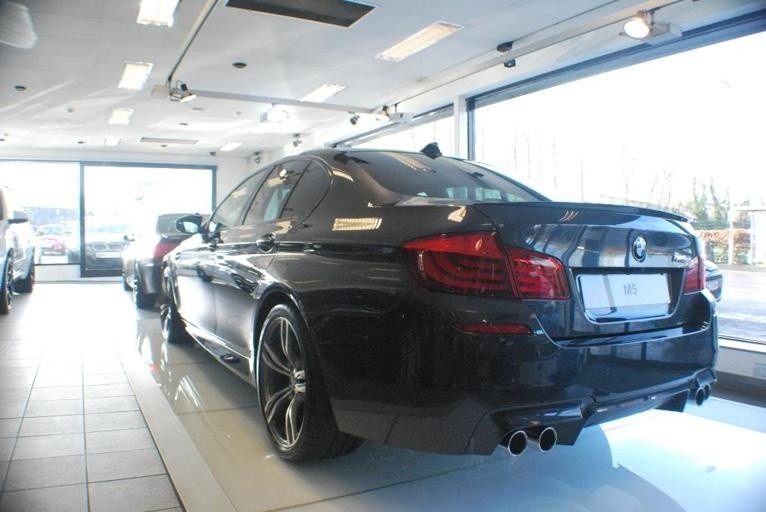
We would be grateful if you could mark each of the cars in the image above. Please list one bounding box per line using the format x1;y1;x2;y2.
157;143;718;463
0;187;36;316
120;212;212;309
33;215;137;277
703;257;723;305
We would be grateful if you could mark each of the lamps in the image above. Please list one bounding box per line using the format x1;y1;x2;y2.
292;135;302;147
622;7;655;41
174;79;197;103
350;111;361;126
253;151;260;164
